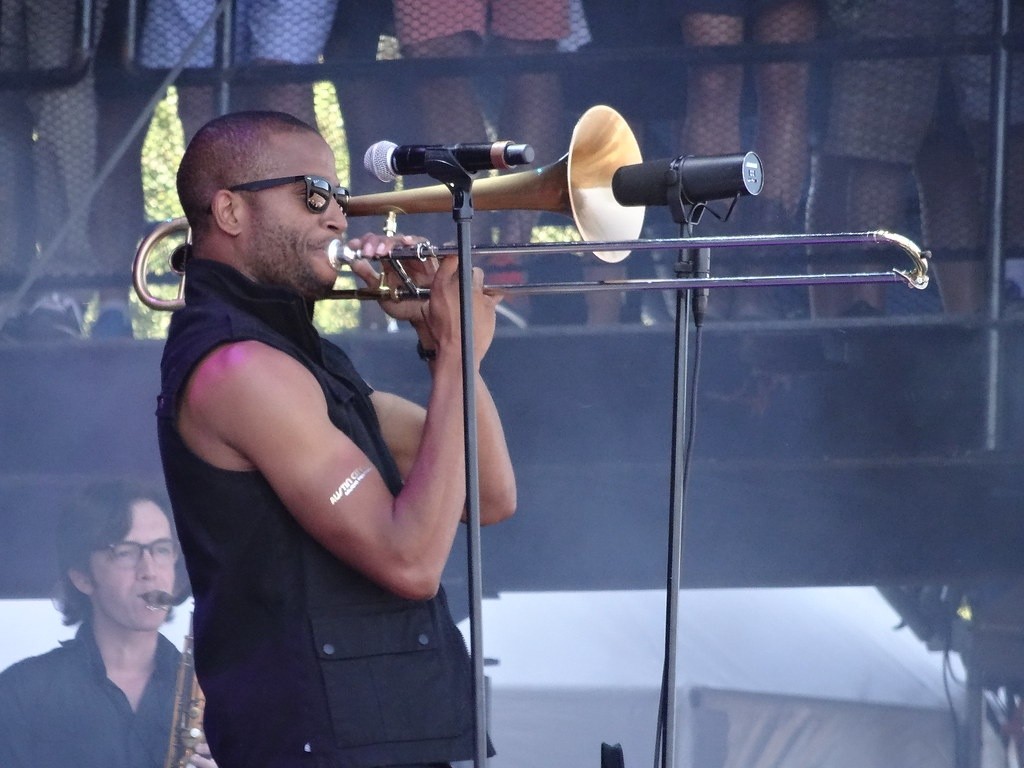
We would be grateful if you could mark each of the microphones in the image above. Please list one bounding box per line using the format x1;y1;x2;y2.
612;151;765;207
364;140;534;183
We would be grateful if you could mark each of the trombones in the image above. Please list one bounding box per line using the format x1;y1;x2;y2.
130;102;935;313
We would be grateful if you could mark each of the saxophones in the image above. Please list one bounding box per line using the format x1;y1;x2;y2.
138;582;211;767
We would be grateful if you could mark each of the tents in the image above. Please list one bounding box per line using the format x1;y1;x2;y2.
0;586;1024;767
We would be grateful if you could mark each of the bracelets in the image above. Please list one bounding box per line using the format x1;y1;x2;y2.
417;342;436;360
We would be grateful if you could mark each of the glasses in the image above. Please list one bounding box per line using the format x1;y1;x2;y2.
204;177;352;223
77;535;182;570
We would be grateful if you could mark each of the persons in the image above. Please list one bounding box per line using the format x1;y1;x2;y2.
153;110;518;767
0;473;217;768
0;0;1024;321
